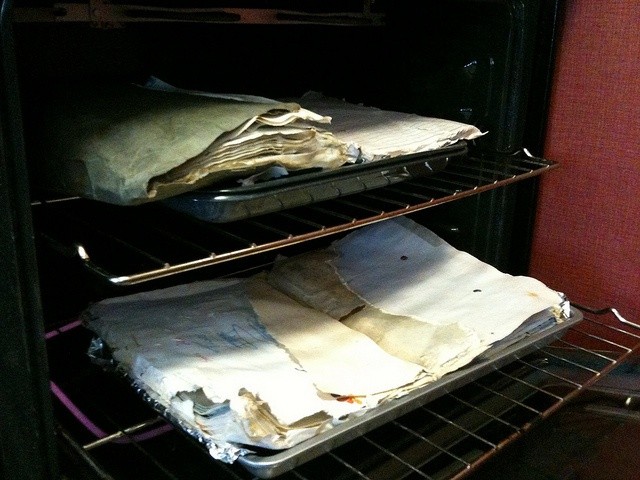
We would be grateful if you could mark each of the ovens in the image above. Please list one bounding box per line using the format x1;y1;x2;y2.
0;2;640;478
